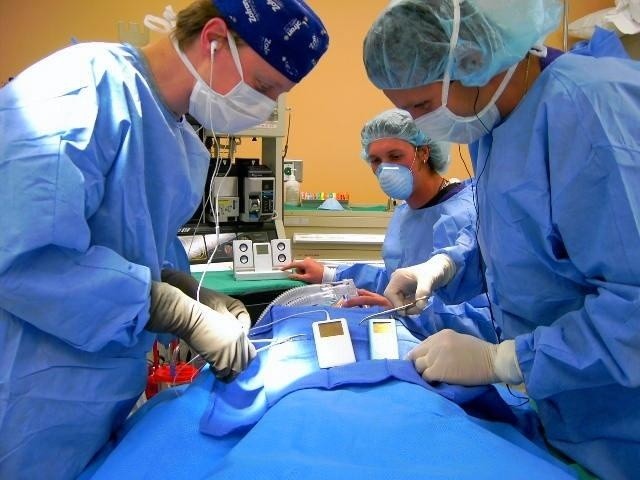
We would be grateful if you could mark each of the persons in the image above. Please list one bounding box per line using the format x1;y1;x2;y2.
280;109;498;343
1;1;329;479
384;1;640;480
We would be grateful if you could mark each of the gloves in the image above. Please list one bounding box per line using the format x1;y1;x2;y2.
384;252;458;315
405;328;524;384
144;269;258;383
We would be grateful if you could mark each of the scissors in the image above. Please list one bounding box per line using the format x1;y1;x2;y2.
246;333;308;353
359;296;430;326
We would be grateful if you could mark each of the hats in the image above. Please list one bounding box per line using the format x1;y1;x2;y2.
214;0;329;83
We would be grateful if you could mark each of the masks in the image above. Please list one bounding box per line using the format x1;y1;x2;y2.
413;55;529;146
188;35;278;135
374;146;419;201
